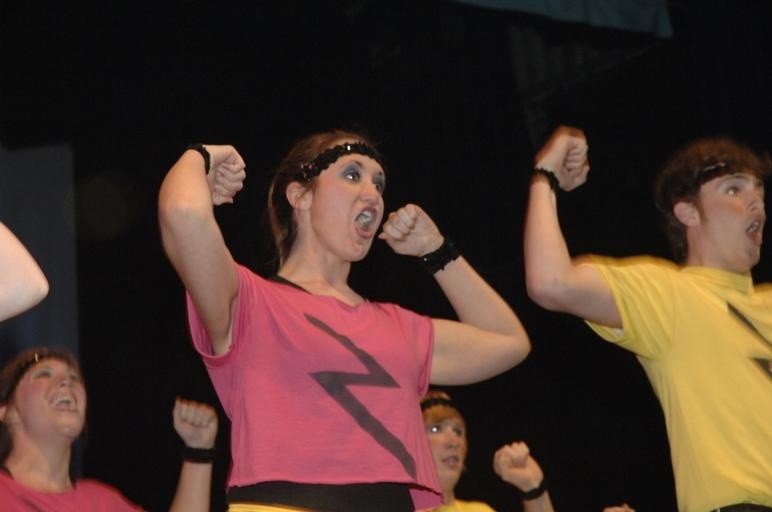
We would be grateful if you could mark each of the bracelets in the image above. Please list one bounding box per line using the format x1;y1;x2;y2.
179;444;221;469
417;238;464;280
529;164;566;196
187;142;214;176
520;479;552;503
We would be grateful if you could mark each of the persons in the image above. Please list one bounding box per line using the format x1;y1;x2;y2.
415;386;555;512
152;126;534;512
1;342;223;512
519;120;771;511
1;220;50;333
